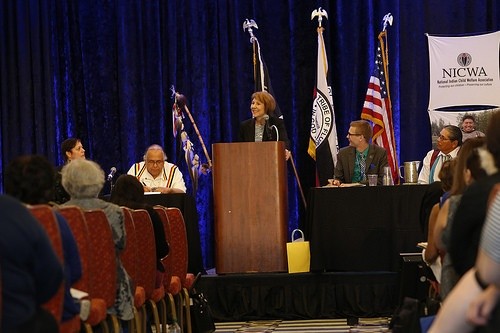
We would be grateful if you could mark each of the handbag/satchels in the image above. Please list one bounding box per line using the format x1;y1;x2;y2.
388;297;443;333
167;273;216;333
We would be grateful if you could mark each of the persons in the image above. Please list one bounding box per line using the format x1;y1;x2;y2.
109;174;170;271
419;125;500;332
3;153;83;320
53;139;87;201
127;145;189;193
0;193;63;333
328;120;387;186
235;92;293;158
61;159;138;319
462;115;485;143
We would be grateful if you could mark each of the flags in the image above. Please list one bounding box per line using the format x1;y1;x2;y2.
360;32;399;186
252;37;290;149
306;26;340;186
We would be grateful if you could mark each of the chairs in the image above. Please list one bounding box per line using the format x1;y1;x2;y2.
25;206;192;333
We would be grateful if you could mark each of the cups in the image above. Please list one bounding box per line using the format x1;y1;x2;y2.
412;161;421;176
399;162;418;183
367;174;378;186
383;166;394;185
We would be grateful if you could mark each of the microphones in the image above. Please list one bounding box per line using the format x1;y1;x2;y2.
269;124;279;141
107;166;117;181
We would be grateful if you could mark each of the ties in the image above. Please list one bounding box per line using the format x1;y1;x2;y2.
359;153;366;183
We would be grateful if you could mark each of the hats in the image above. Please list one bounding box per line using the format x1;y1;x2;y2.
113;174;145;202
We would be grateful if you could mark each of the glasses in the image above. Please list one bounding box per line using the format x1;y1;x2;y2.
348;131;361;136
147;160;164;165
438;134;449;140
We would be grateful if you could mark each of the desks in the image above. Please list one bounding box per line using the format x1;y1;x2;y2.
311;181;443;271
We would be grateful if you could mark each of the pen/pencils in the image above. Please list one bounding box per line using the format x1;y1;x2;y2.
333;174;337;186
141;181;152;190
